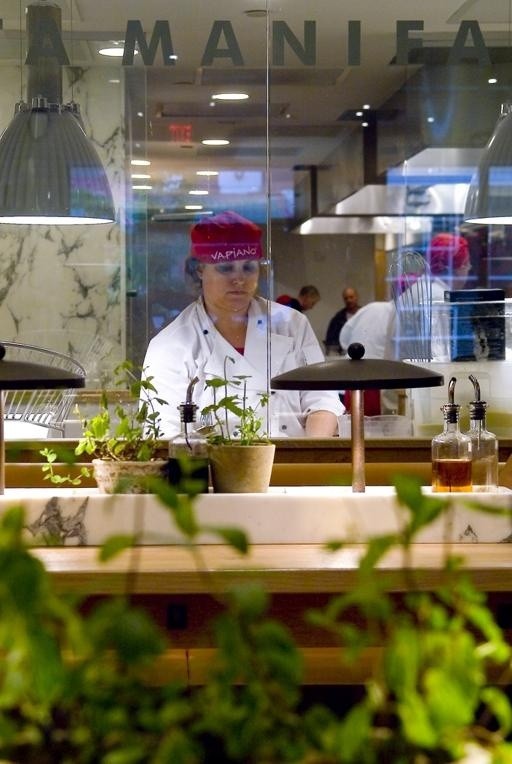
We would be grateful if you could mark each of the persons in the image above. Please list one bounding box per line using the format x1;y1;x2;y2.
323;287;364;356
386;234;472;413
277;286;321;311
338;272;420;413
137;211;347;441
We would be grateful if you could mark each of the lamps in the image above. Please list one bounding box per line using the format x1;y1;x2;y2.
0;1;115;225
463;103;512;226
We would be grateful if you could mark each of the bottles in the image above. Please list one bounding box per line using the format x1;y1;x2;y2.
463;374;499;494
428;377;474;494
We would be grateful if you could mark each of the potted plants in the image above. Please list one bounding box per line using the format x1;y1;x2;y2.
196;356;276;493
39;360;169;493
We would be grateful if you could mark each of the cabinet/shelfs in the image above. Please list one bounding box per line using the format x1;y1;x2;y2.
0;463;512;727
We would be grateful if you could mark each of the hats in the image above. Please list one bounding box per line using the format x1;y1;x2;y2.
192;212;263;264
427;234;468;273
